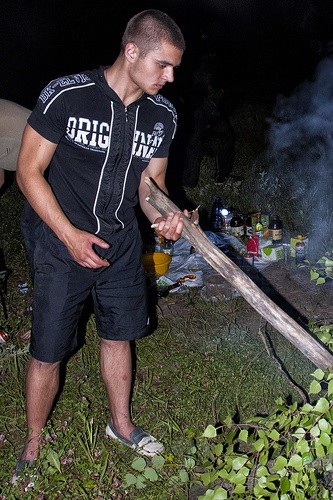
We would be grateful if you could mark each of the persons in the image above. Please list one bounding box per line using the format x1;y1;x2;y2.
11;9;191;486
178;28;245;190
0;98;32;187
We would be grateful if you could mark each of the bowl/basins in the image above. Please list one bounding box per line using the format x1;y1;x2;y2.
143;252;172;277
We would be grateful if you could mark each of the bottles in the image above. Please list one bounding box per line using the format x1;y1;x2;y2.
158;235;174;257
295;235;305;265
213;206;284;257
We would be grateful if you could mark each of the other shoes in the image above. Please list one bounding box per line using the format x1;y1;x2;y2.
104;423;165;457
10;459;36;492
